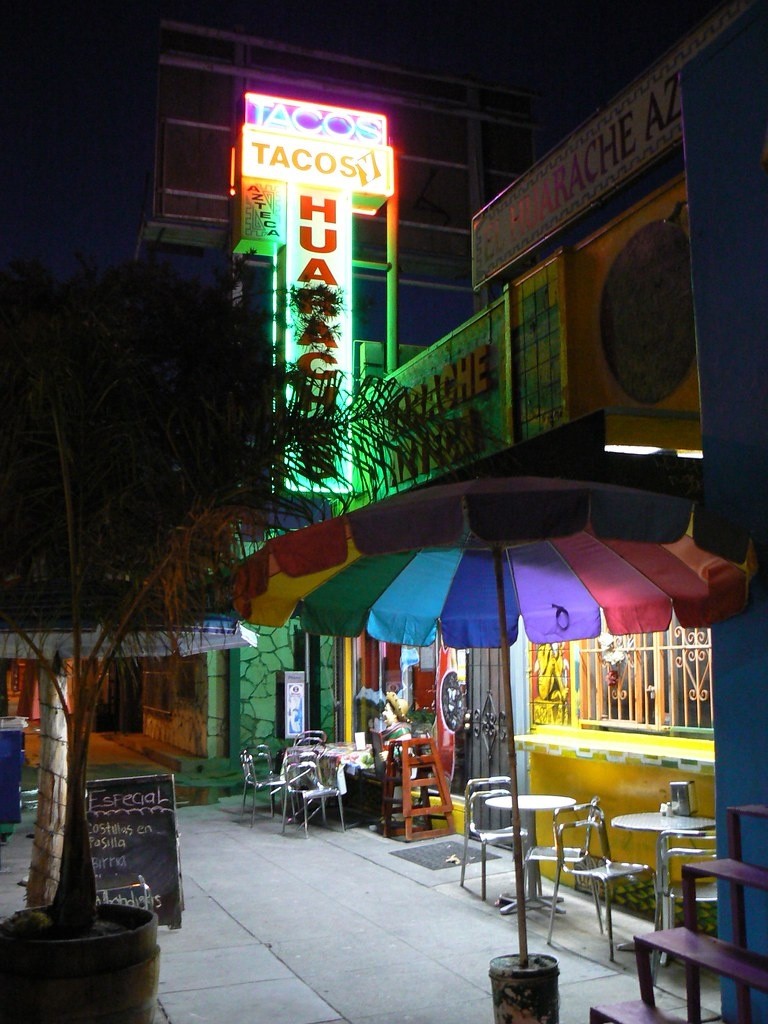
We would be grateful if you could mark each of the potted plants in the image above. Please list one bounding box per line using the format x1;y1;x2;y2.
0;306;511;1024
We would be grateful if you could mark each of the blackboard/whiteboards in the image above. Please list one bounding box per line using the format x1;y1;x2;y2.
85;774;184;925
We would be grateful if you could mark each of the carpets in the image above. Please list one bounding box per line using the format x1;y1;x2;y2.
389;841;502;871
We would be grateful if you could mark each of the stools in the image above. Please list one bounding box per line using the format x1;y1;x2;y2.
382;739;456;842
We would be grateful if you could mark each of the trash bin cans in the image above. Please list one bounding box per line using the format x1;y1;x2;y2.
0;730;25;823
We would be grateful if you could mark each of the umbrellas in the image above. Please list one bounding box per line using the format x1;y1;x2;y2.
227;472;763;1024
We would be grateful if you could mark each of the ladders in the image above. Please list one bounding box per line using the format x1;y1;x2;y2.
380;738;456;843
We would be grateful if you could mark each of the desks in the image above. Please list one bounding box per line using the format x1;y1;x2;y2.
610;811;715;966
484;795;576;917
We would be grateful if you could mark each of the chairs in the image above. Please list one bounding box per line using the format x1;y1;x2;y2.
523;796;602;911
459;776;531;902
650;829;717;983
545;804;659;960
239;733;346;840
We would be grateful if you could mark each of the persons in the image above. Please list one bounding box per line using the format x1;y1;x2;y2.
366;692;419;781
